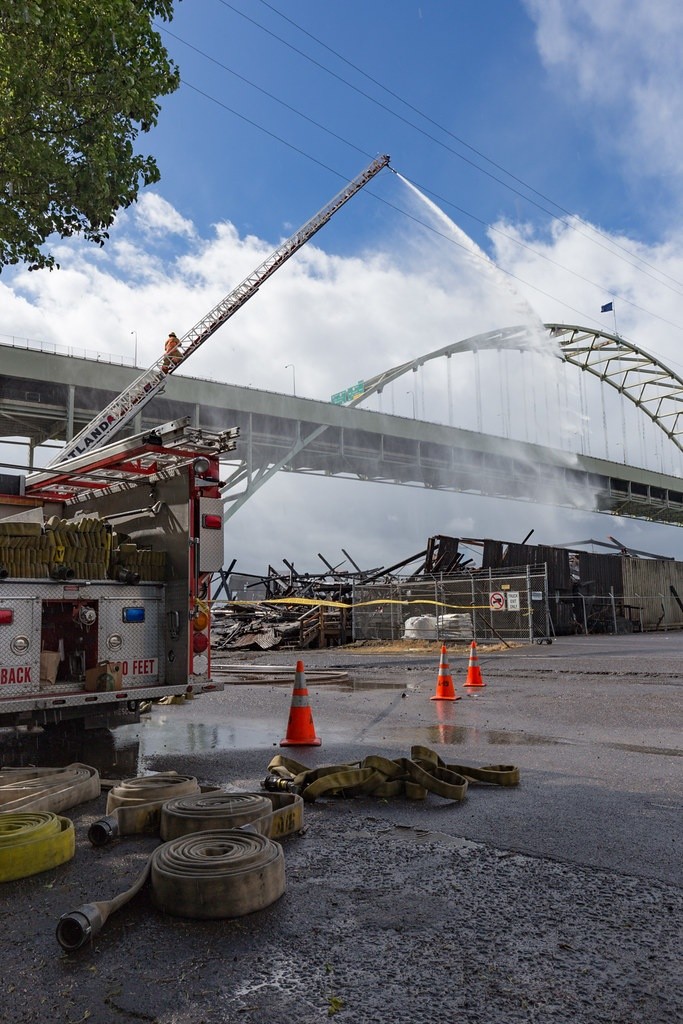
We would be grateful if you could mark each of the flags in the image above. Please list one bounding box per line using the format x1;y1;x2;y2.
601;302;613;313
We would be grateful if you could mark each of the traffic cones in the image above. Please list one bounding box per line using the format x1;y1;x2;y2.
279;659;323;746
429;644;462;700
462;641;487;686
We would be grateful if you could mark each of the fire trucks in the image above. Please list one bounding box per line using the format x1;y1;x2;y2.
0;152;395;727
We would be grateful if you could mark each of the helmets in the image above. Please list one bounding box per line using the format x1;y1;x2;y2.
169;332;176;338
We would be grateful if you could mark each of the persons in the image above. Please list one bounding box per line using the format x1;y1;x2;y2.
161;332;183;374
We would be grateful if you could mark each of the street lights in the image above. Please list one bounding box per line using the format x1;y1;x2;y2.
130;330;137;367
407;390;415;419
285;364;296;395
